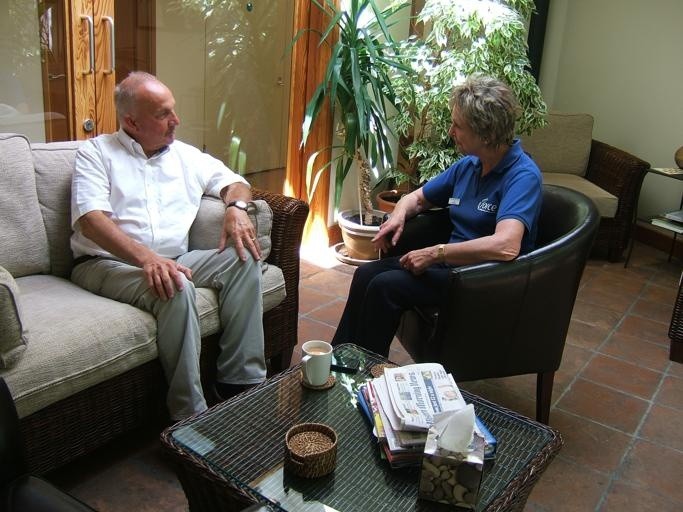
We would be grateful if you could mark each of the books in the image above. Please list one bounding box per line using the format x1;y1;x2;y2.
357;365;498;470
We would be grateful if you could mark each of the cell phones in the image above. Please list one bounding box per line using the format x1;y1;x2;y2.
332;353;360;373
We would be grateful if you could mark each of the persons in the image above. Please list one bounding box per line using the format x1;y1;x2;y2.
70;67;267;426
330;77;542;361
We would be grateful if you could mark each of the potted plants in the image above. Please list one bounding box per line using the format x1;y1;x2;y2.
376;0;543;210
280;0;419;263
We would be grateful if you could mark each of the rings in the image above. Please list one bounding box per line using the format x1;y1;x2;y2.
252;237;257;242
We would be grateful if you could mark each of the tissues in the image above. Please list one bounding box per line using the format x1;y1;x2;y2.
418;403;486;509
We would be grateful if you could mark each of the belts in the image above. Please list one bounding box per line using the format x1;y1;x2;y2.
72;254;98;267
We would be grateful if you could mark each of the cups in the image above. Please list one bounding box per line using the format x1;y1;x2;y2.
299;340;332;385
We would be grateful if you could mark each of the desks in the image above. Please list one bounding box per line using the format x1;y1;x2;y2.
622;167;683;267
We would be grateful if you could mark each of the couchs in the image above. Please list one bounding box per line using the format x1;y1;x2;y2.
0;132;309;476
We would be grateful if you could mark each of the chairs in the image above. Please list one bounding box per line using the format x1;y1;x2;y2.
512;103;651;264
379;184;600;427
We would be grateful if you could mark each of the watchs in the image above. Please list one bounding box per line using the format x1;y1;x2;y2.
225;200;250;211
438;242;449;265
